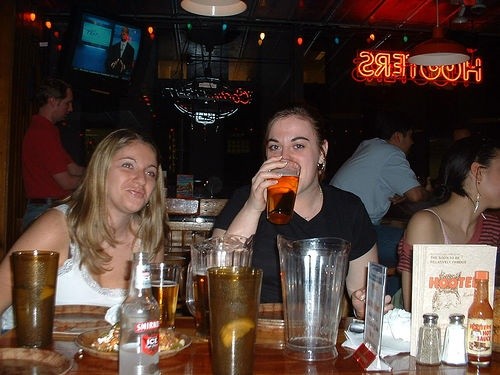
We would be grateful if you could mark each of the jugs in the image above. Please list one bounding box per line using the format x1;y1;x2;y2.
185;232;255;343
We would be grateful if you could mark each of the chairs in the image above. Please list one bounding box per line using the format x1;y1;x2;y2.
164;197;232;315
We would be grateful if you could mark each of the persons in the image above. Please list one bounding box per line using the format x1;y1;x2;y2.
0;128;173;332
105;26;134;76
21;77;87;234
327;123;432;268
208;105;393;320
427;128;472;171
397;134;500;312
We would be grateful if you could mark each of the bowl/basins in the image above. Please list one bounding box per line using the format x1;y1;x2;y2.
256;302;286;350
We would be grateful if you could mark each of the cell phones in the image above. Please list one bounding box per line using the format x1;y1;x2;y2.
345;317;365;333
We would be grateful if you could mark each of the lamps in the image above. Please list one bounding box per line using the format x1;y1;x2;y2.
180;0;247;18
408;0;488;66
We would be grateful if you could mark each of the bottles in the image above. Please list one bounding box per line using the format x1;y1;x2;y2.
442;313;469;366
465;270;494;366
415;312;442;366
118;252;161;375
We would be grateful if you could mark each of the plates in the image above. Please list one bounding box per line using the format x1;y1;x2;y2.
74;326;191;362
0;347;73;375
52;304;111;342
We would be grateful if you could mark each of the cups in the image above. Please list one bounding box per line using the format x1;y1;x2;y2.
207;266;264;375
149;262;182;332
265;158;301;224
12;251;59;350
276;234;351;363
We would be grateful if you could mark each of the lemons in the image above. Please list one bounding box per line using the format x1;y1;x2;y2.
39;286;53;300
220;318;254;347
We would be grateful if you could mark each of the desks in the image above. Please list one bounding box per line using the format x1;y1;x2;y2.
0;317;500;375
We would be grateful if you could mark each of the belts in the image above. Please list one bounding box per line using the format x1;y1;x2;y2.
26;198;58;204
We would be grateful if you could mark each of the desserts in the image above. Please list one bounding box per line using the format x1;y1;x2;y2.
91;324;178;351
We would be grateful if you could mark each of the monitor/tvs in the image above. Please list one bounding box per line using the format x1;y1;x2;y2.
72;14;144;86
